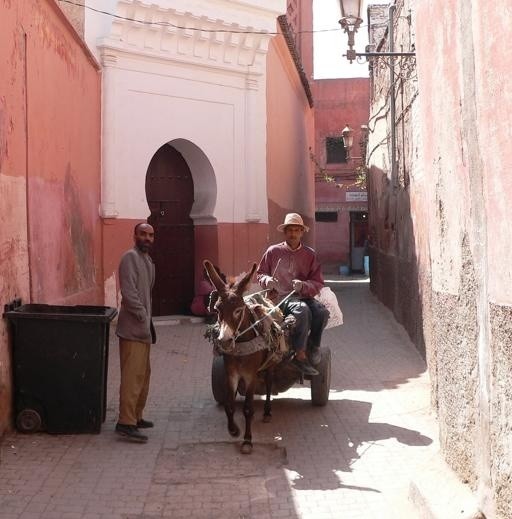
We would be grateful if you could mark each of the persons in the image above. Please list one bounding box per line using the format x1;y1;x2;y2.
115;223;157;443
255;214;325;376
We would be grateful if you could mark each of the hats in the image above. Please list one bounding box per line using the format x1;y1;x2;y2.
277;212;310;233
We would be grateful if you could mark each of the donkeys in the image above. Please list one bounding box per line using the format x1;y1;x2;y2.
202;259;291;454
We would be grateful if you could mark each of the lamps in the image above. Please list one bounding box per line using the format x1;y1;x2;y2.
341;122;364;160
339;0;416;64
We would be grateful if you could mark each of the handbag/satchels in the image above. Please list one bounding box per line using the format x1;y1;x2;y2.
312;279;345;330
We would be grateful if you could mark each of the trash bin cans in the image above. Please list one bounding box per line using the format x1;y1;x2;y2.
2;305;118;434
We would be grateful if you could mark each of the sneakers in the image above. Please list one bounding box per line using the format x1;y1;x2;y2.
287;346;322;376
115;418;154;441
